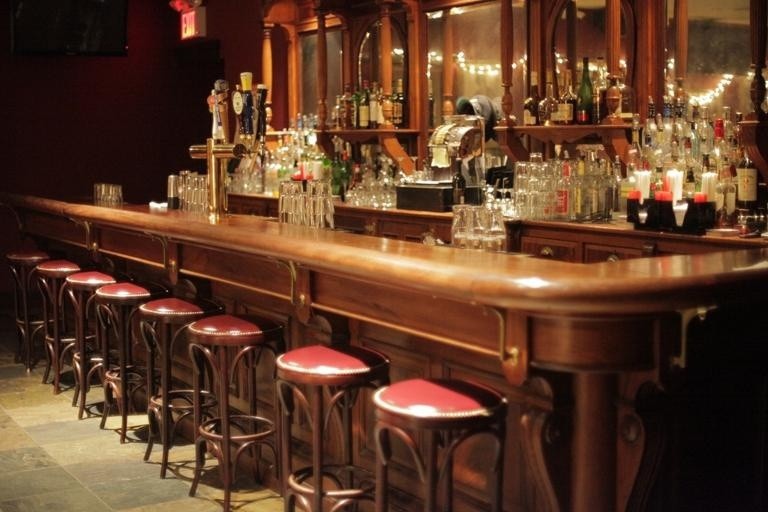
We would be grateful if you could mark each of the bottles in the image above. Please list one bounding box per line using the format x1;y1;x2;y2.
557;70;576;125
368;81;380;129
573;161;593;221
576;57;592;125
736;148;759;211
555;162;576;223
589;149;605;221
268;112;327;199
330;151;340;196
523;85;538;126
357;79;370;130
351;87;359;130
393;78;404;129
621;98;743;225
340;86;349;130
340;151;349;198
592;57;607;125
538;81;557;126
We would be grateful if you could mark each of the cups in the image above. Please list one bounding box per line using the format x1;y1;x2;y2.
511;153;569;220
451;203;508;251
278;180;336;230
178;171;210;213
93;184;124;208
346;171;397;212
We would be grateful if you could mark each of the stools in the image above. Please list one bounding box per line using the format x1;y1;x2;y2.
187;312;282;512
138;296;226;479
59;270;118;421
77;282;158;445
371;376;508;512
4;251;50;363
24;259;80;383
274;342;390;512
